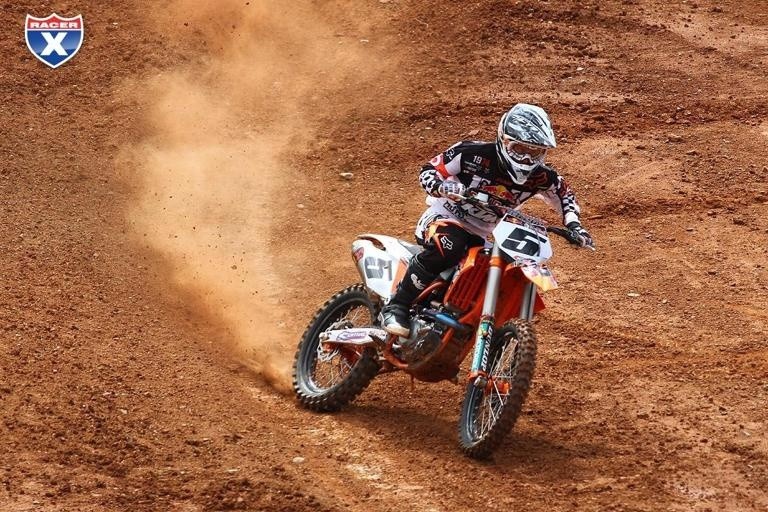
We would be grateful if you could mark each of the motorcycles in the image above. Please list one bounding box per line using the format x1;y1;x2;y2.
292;187;594;456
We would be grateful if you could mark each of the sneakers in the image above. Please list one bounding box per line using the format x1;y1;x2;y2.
377;306;410;337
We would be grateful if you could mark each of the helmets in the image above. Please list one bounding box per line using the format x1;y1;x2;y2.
495;102;557;185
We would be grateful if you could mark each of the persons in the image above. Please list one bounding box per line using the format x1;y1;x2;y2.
377;103;592;337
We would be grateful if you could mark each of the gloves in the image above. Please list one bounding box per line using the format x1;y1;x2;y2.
571;226;592;247
439;180;466;202
